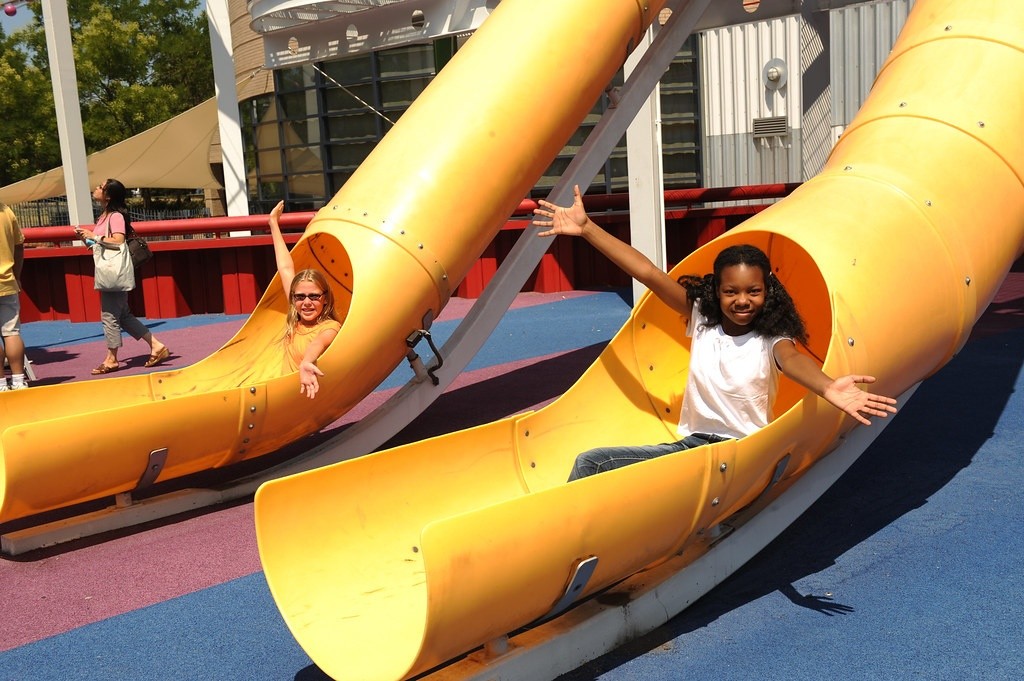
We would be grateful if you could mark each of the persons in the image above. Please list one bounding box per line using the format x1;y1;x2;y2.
0;202;30;392
531;184;898;483
268;200;342;400
74;179;172;375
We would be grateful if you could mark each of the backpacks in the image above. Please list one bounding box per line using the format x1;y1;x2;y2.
109;211;153;267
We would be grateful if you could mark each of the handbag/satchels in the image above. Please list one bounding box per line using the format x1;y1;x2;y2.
92;211;136;291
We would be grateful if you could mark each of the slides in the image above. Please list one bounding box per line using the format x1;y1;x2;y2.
251;2;1021;681
0;0;665;528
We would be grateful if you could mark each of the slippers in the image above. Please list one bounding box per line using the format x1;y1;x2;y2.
91;363;120;375
144;348;170;366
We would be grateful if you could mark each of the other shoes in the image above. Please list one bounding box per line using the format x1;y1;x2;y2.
0;382;28;391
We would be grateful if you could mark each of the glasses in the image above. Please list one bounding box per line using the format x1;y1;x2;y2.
100;185;107;192
293;293;325;302
